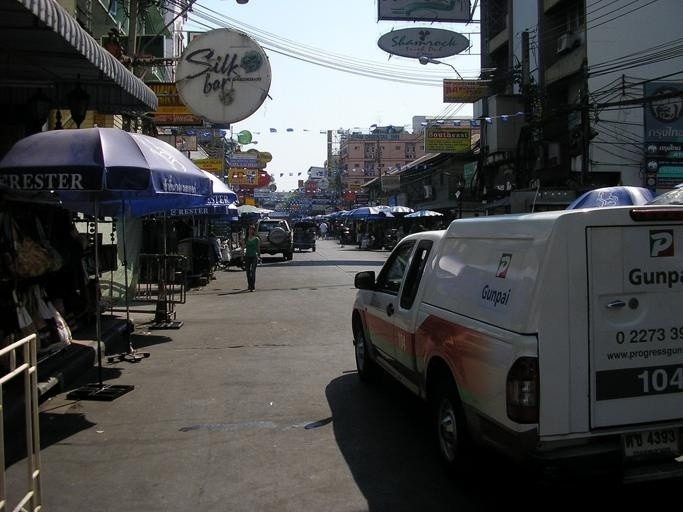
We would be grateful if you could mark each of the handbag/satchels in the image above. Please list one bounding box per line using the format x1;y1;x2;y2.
14;284;73;353
8;213;49;277
35;217;66;272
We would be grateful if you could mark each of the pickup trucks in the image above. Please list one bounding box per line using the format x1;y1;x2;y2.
347;205;682;485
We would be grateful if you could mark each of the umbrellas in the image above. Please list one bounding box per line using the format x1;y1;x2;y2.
565;185;654;210
302;205;444;230
645;183;683;205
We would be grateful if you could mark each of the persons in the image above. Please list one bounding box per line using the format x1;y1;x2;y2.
319;221;329;240
243;225;261;292
215;236;257;269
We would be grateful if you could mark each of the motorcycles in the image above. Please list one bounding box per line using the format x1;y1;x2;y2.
216;239;247;271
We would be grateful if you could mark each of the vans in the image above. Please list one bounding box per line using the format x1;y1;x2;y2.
291;222;317;252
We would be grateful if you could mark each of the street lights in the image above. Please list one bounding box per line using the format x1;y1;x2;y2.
134;1;250;74
418;57;464;81
369;123;384;194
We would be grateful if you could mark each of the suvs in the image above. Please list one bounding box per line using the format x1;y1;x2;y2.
255;216;294;261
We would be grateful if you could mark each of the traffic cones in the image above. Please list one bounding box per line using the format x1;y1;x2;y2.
154;280;170;323
